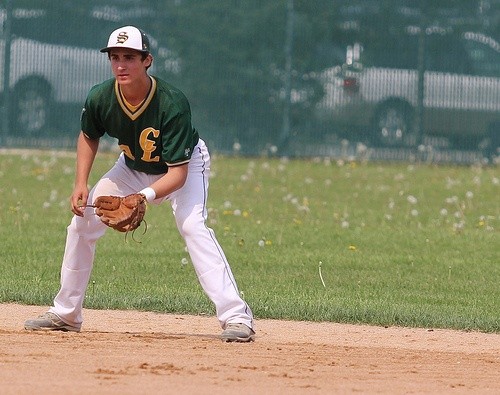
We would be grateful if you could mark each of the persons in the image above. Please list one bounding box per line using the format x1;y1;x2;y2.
479;112;499;158
24;26;256;342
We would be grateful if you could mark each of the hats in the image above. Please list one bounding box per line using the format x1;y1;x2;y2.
100;25;150;53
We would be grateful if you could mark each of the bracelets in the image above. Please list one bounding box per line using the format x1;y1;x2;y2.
136;187;156;204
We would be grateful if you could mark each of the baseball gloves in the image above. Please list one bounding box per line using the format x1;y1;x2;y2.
93;193;148;244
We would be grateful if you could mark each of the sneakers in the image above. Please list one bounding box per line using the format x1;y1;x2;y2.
221;324;255;341
24;312;81;332
